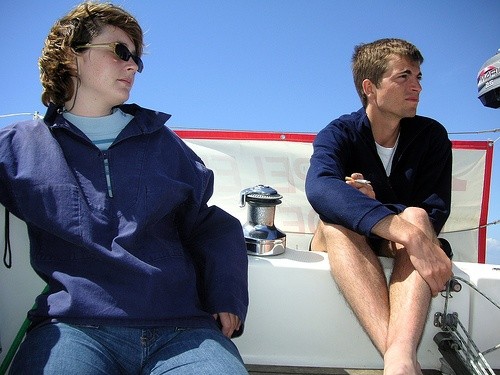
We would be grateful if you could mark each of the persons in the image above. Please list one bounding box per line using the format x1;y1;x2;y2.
0;1;248;375
305;39;453;375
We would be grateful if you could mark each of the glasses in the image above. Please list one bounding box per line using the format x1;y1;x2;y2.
75;41;144;73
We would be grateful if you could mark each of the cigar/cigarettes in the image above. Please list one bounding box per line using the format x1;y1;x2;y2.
345;176;371;184
